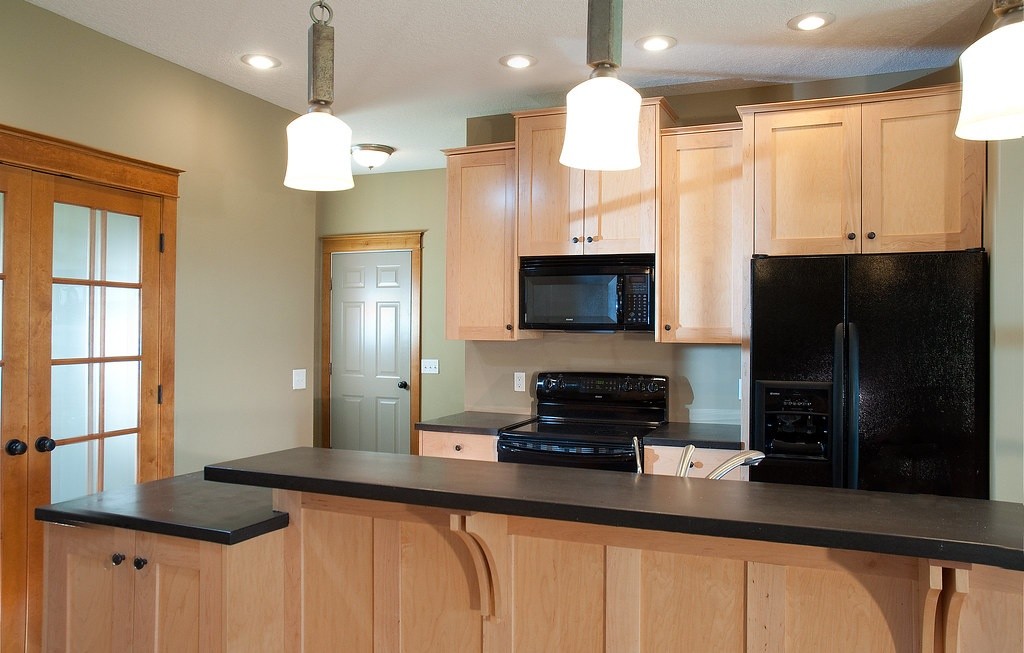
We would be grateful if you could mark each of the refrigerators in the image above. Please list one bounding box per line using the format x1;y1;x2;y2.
749;247;990;501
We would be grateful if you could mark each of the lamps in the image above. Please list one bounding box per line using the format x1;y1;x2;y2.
283;1;354;191
351;144;394;170
558;0;641;171
955;0;1024;142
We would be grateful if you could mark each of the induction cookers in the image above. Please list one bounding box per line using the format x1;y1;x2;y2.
501;371;669;449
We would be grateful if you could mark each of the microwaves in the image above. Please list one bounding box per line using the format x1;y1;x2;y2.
518;253;656;333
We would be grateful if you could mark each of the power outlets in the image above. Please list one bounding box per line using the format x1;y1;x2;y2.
514;372;525;392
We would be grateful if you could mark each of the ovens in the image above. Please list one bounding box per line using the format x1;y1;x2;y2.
497;440;643;474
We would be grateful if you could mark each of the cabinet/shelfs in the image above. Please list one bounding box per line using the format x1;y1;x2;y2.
417;430;499;463
41;522;286;653
441;82;987;344
644;445;741;482
